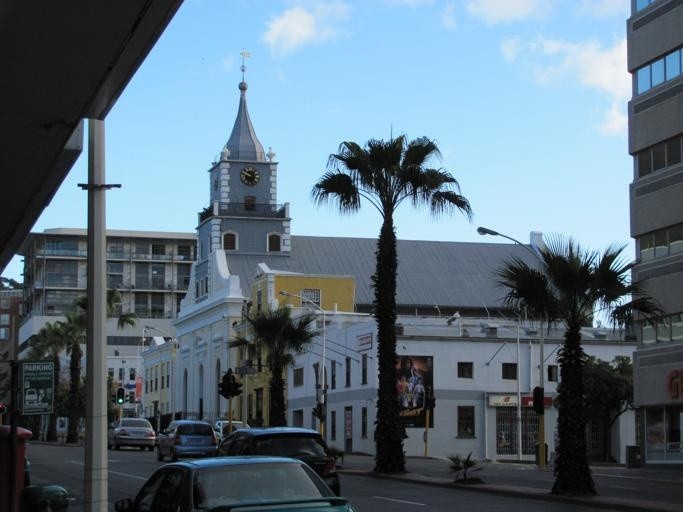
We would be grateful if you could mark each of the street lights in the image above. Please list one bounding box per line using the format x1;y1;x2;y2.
145;325;176;421
477;226;545;471
218;374;243;399
279;290;327;438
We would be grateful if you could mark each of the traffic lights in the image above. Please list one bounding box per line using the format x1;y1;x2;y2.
112;387;129;405
417;392;436;409
312;403;326;418
532;386;544;416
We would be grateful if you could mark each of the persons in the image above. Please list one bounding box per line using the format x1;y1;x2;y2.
393;355;425;409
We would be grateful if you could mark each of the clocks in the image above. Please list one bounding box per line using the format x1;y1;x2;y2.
240;167;260;186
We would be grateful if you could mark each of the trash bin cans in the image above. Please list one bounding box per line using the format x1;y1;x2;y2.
21;484;69;512
535;443;549;466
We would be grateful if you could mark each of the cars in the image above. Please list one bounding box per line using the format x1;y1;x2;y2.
107;417;358;511
